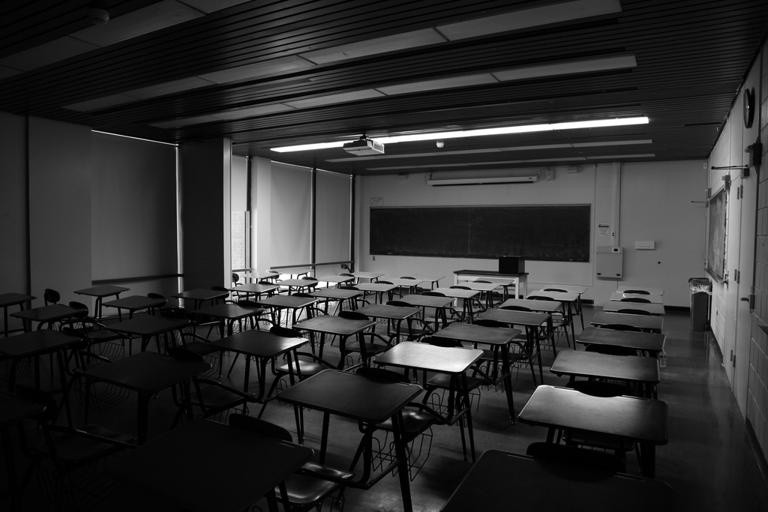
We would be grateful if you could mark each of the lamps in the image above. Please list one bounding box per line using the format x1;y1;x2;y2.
425;169;540;187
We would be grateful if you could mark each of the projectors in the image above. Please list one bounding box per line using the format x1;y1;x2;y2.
342;138;385;157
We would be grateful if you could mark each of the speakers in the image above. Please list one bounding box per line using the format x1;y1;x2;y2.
499;256;526;274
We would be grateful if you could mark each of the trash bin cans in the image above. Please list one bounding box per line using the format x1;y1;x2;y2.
690;279;713;332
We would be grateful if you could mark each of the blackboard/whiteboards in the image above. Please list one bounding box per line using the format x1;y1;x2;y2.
369;204;591;262
